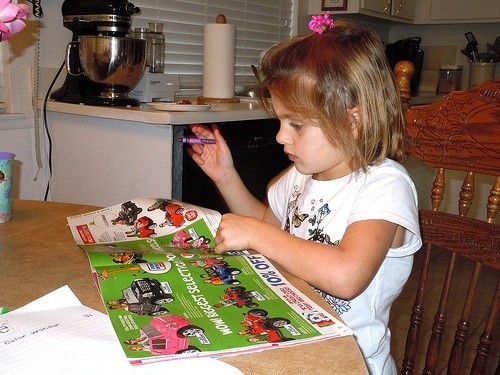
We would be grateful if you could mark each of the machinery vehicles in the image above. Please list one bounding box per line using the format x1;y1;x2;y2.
146;198;188;228
110;200;159;239
95;249;206;357
163;230;293;344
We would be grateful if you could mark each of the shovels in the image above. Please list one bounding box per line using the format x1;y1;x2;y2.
464;32;480;62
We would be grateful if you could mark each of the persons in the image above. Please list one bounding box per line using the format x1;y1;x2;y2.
192;13;422;375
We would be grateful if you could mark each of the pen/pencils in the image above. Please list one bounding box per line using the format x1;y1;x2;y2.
177;138;216;144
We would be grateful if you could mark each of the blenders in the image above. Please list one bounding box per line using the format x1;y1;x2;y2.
49;0;148;107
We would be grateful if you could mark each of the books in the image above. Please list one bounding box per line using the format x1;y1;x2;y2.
66;197;353;366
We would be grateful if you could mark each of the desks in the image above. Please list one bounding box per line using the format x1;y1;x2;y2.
0;198;370;375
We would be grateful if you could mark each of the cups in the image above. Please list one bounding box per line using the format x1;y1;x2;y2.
127;22;165;73
438;68;463;94
0;151;16;224
468;62;496;89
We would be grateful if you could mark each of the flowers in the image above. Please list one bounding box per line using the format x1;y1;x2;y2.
0;0;31;42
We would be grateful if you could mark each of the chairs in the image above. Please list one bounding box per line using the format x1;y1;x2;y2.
394;60;500;375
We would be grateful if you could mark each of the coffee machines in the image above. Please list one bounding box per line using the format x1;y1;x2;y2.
385;35;424;96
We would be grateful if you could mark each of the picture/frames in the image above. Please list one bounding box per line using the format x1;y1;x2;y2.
321;0;347;11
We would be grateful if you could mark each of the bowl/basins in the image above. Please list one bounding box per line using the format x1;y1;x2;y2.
66;34;148;97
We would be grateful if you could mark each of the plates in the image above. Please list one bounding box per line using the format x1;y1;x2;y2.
146;102;213;112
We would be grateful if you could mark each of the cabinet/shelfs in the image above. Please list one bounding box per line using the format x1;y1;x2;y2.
414;0;500;24
309;0;414;24
